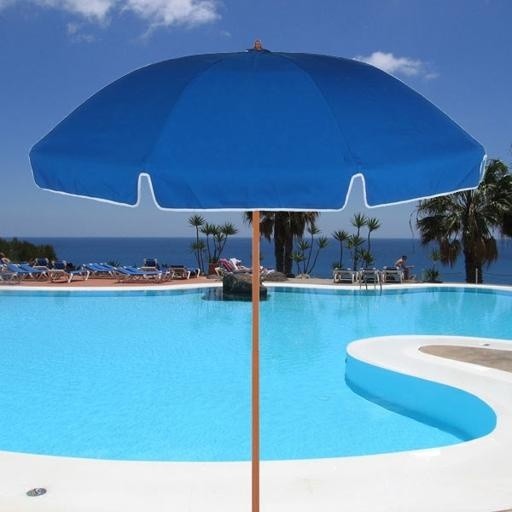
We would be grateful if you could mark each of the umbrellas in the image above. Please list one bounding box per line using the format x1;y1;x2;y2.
25;40;494;512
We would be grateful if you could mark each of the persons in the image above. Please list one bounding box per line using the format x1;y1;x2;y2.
393;253;416;280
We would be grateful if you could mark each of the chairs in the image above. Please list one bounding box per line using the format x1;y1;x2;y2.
334;266;403;284
216;258;267;279
1;255;201;284
